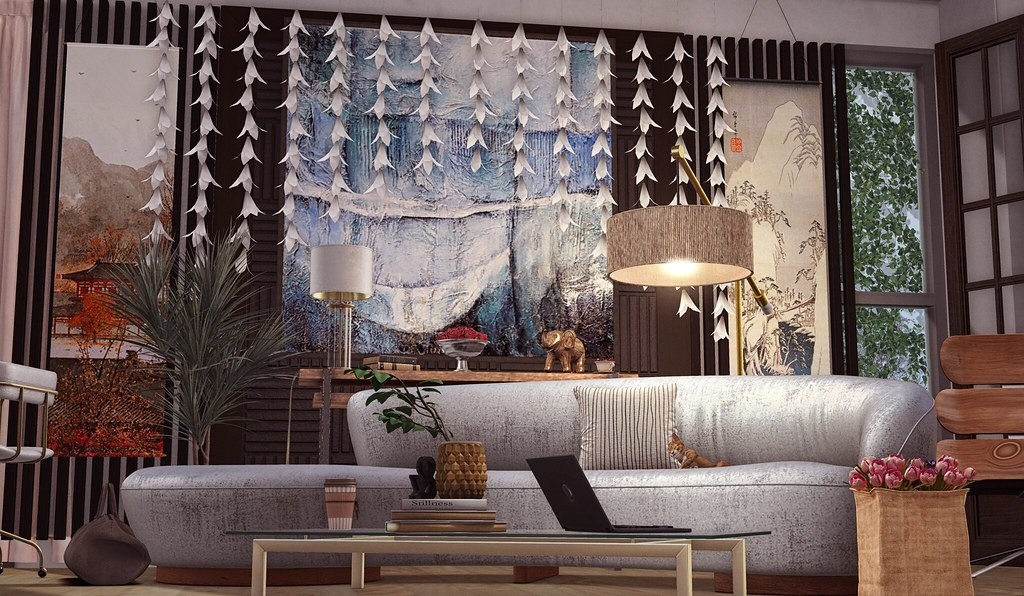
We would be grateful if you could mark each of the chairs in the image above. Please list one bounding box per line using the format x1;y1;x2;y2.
0;361;59;578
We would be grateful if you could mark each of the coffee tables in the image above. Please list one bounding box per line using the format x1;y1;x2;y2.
225;529;772;596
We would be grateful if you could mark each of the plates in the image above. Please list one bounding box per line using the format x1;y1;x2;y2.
592;372;614;374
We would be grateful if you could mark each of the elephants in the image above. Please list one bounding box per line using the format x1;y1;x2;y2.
539;321;585;374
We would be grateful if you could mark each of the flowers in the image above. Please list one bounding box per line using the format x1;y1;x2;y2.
842;453;978;492
435;326;489;341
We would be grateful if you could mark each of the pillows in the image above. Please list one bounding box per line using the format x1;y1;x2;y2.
573;382;681;470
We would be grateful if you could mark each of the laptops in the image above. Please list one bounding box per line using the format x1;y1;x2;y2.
526;455;693;533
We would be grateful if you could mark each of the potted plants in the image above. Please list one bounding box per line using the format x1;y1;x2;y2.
343;364;488;499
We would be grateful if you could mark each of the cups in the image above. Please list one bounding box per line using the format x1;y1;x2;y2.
594;361;615;372
324;478;356;531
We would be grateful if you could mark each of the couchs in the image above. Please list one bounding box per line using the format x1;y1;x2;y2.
116;376;938;596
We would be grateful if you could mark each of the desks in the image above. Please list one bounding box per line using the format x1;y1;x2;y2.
297;368;639;464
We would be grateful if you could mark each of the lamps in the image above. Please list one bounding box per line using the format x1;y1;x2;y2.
309;244;373;369
605;145;773;375
